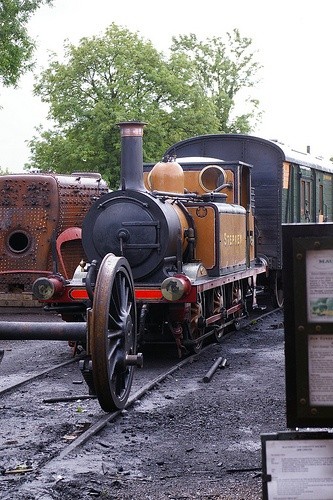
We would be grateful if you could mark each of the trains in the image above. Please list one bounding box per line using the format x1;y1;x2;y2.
0;121;333;413
